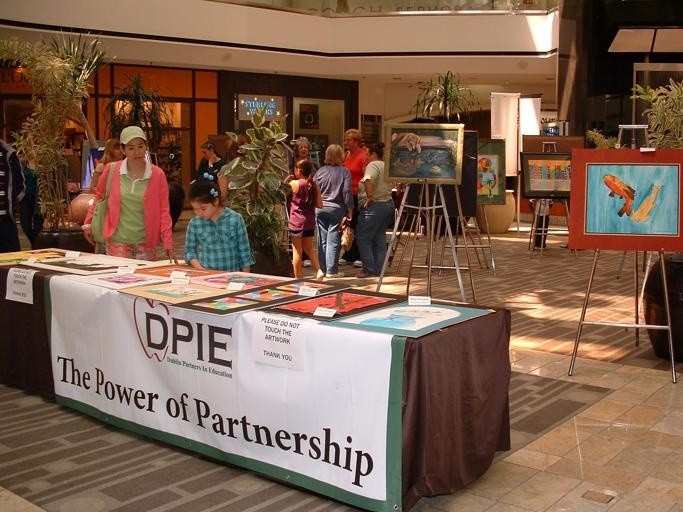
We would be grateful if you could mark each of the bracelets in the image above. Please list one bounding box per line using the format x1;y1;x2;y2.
81;224;92;231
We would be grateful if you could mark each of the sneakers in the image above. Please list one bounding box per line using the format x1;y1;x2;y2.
303;260;311;267
316;269;323;281
353;261;362;267
338;258;353;265
326;272;345;278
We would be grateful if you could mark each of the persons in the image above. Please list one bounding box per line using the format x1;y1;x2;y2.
195;141;225;182
82;125;177;265
88;138;123;188
529;198;552;250
284;129;395;283
184;180;257;272
0;138;27;253
20;158;40;249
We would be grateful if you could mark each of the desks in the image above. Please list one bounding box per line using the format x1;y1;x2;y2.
0;248;511;512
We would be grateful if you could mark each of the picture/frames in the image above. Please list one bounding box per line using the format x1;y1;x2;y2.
518;151;570;198
384;122;466;185
570;147;683;253
476;137;506;205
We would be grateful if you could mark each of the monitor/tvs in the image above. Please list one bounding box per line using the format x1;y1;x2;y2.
79;139;106;189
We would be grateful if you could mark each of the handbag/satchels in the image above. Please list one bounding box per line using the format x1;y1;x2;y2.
340;225;354;252
90;198;107;244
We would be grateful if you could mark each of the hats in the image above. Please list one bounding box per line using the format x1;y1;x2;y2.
118;126;147;145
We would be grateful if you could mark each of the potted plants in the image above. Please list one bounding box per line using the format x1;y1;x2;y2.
0;28;104;252
628;80;683;361
216;100;295;278
